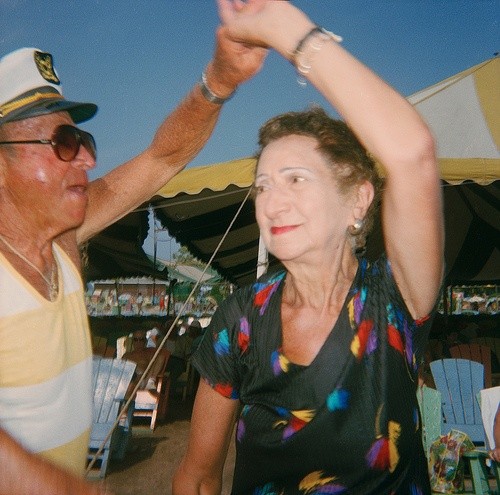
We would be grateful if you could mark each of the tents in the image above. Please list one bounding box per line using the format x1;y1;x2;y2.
148;55;499;336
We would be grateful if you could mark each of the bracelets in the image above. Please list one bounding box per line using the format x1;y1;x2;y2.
289;26;343;89
199;67;238;105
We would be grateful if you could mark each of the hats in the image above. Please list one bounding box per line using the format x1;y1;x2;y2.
2;48;98;123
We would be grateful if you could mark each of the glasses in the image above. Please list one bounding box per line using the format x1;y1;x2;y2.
2;125;97;161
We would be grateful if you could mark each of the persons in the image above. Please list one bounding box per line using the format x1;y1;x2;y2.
171;0;445;495
487;402;500;464
181;321;203;365
120;330;163;400
0;25;271;495
156;323;187;398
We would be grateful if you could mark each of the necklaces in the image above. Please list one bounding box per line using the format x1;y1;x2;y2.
0;234;59;303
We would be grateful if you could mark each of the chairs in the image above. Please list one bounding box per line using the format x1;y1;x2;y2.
450;343;492;391
429;359;485;449
84;360;136;478
122;348;171;428
414;386;493;495
92;335;114;356
476;388;500;475
